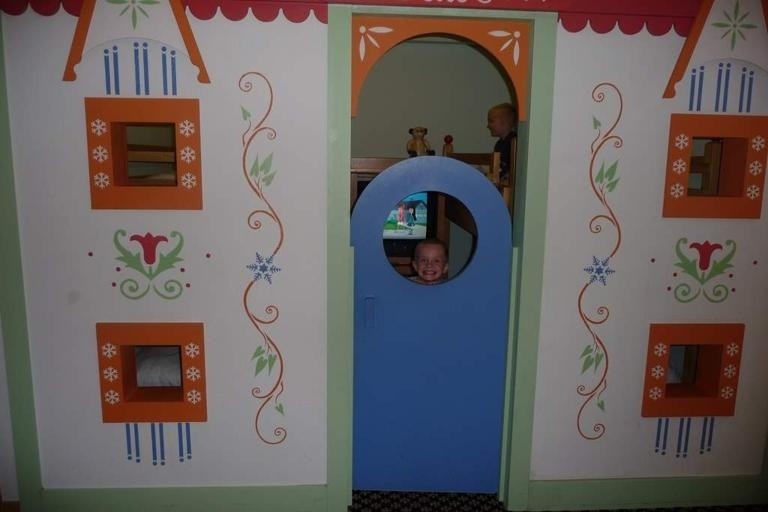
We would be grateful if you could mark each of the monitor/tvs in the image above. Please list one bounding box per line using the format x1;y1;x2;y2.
357;180;437;250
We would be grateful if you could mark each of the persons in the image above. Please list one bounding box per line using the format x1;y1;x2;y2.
443;134;455;155
407;207;416;227
485;101;516;185
403;238;452;286
404;127;436;157
398;204;406;222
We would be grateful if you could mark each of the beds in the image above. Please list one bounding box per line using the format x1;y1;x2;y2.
437;134;516;280
